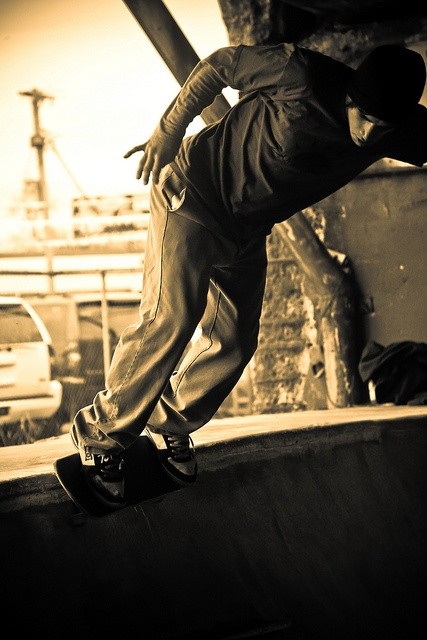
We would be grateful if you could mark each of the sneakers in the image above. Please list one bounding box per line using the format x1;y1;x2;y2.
144;424;199;489
70;423;128;510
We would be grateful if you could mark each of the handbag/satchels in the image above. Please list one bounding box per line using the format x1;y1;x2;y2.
358;340;427;404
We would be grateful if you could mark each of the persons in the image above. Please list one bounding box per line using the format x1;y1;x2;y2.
72;39;427;512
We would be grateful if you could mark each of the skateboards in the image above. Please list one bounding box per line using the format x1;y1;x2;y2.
54;435;199;516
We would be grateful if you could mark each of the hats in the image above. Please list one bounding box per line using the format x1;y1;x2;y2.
347;44;426;124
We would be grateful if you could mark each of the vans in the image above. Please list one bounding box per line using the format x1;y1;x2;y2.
0;297;62;423
